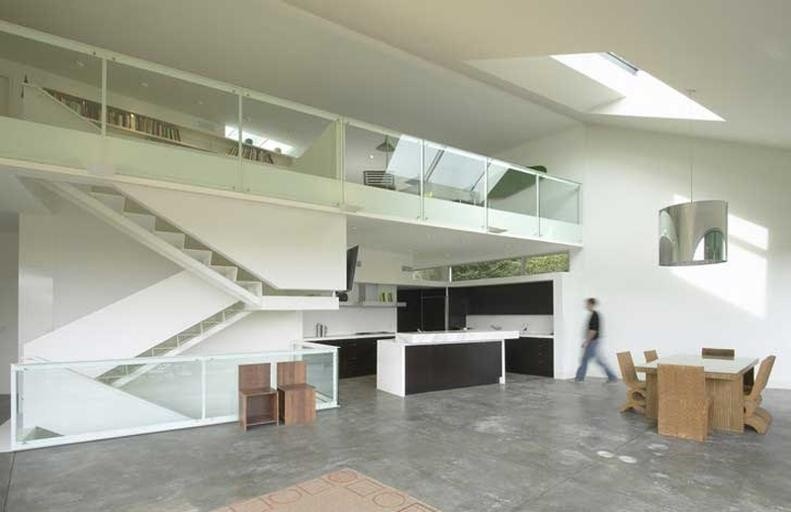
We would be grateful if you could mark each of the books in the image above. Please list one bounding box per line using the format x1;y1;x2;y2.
55;94;181;141
229;143;274;163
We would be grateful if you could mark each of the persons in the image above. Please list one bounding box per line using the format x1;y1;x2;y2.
571;298;616;385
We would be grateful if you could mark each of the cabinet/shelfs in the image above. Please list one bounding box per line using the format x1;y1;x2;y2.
239;361;315;432
397;289;465;331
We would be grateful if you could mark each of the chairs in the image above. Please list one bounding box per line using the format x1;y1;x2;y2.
616;347;776;443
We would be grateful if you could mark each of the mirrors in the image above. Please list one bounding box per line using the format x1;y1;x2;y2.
659;201;727;265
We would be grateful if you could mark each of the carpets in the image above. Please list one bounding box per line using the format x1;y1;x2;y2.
203;467;441;511
